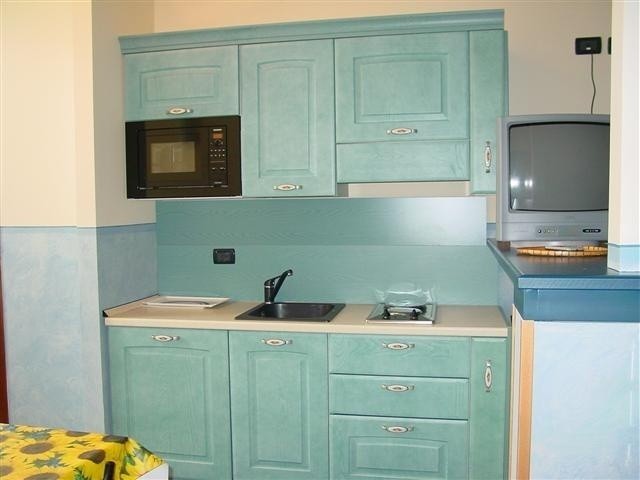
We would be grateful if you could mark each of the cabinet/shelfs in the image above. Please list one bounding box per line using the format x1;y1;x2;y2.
469;29;504;195
328;334;471;480
124;44;240;123
228;331;329;480
469;338;509;480
335;31;472;184
238;38;337;198
107;327;233;480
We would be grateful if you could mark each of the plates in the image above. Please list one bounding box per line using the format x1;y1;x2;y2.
142;295;231;310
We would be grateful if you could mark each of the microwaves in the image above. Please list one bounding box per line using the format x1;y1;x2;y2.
124;114;243;200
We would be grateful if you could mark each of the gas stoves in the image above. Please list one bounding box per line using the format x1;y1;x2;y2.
364;297;439;325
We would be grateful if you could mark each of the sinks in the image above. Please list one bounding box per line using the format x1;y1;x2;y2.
236;302;345;322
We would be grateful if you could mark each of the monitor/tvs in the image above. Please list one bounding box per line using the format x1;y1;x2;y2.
496;112;611;252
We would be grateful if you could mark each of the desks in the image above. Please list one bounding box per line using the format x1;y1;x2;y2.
0;423;169;480
486;238;640;480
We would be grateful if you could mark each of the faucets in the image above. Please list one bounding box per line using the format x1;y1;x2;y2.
264;267;294;305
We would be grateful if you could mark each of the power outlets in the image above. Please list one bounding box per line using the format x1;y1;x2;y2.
213;248;235;264
575;37;602;55
